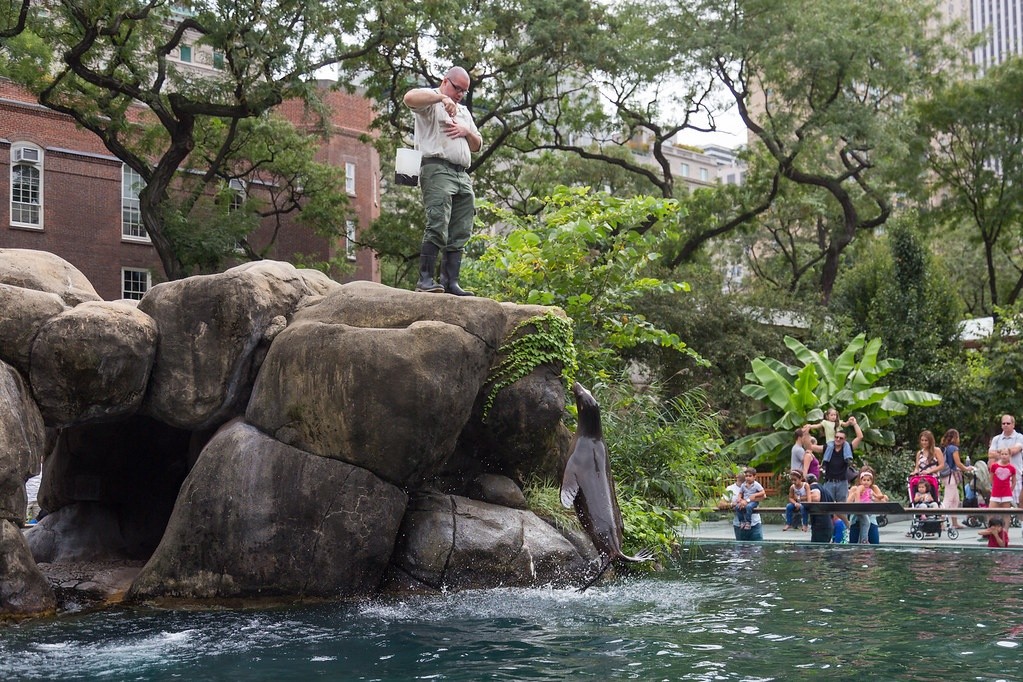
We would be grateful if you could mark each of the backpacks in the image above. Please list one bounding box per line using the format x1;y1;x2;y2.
939;446;957;477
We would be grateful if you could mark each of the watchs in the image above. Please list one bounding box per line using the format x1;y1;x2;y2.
746;498;750;502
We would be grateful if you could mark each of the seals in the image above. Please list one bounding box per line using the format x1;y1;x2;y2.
559;380;657;595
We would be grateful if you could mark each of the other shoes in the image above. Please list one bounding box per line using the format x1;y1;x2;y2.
783;525;793;531
1017;520;1023;527
740;521;745;529
822;464;826;469
940;526;948;532
906;531;912;537
921;515;927;520
952;524;964;529
977;537;989;542
861;540;870;544
925;533;933;536
745;522;751;530
847;459;853;464
802;526;808;532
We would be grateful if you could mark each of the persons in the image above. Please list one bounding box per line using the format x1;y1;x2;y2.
847;467;886;544
403;67;483;296
989;448;1016;531
791;429;819;479
855;471;888;544
978;517;1009;548
795;473;850;542
940;429;972;530
802;409;863;520
910;431;944;536
988;415;1023;527
783;469;811;532
718;467;765;540
914;479;939;520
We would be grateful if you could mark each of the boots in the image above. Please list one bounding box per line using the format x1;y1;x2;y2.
440;250;474;296
414;241;445;293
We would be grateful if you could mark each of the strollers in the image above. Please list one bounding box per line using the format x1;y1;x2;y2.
906;474;960;542
821;466;889;528
962;469;1023;529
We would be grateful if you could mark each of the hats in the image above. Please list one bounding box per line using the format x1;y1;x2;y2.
806;473;817;481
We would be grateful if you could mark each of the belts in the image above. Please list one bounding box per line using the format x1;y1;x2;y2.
421;157;465;172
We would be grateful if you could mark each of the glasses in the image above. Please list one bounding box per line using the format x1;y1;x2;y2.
446;77;469;95
1002;422;1013;425
835;435;845;439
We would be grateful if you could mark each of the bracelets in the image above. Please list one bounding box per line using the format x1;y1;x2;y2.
797;498;800;502
